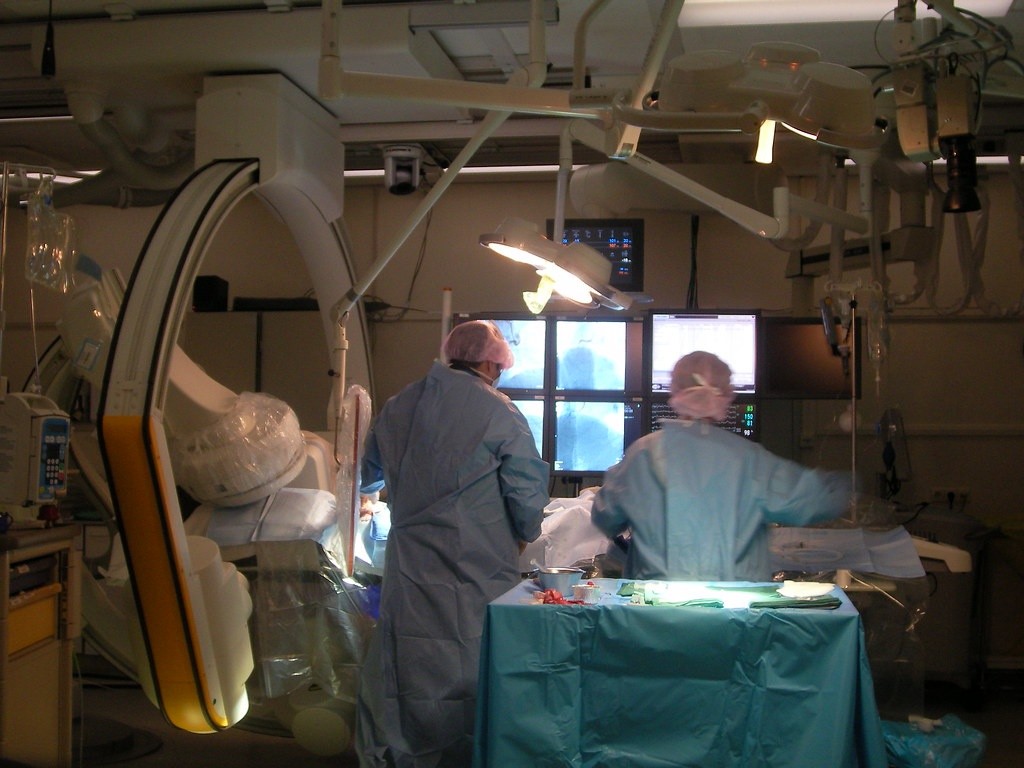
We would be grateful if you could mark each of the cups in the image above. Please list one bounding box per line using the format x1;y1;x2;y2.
572;584;601;604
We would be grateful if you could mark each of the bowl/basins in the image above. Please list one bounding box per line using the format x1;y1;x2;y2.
538;567;586;597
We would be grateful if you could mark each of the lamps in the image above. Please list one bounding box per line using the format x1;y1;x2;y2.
611;41;891;164
481;133;636;314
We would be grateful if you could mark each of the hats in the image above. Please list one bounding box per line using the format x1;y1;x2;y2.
443;319;514;369
667;350;734;423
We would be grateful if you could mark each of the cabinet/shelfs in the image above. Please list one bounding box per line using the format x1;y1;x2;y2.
0;523;83;768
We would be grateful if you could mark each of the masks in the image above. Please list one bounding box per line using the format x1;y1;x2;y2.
469;366;501;390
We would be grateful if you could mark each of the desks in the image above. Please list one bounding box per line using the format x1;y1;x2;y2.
472;578;889;768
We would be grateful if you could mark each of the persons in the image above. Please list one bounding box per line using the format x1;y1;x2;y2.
359;322;549;768
591;351;862;583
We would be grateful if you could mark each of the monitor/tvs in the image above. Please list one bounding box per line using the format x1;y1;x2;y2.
450;307;764;476
